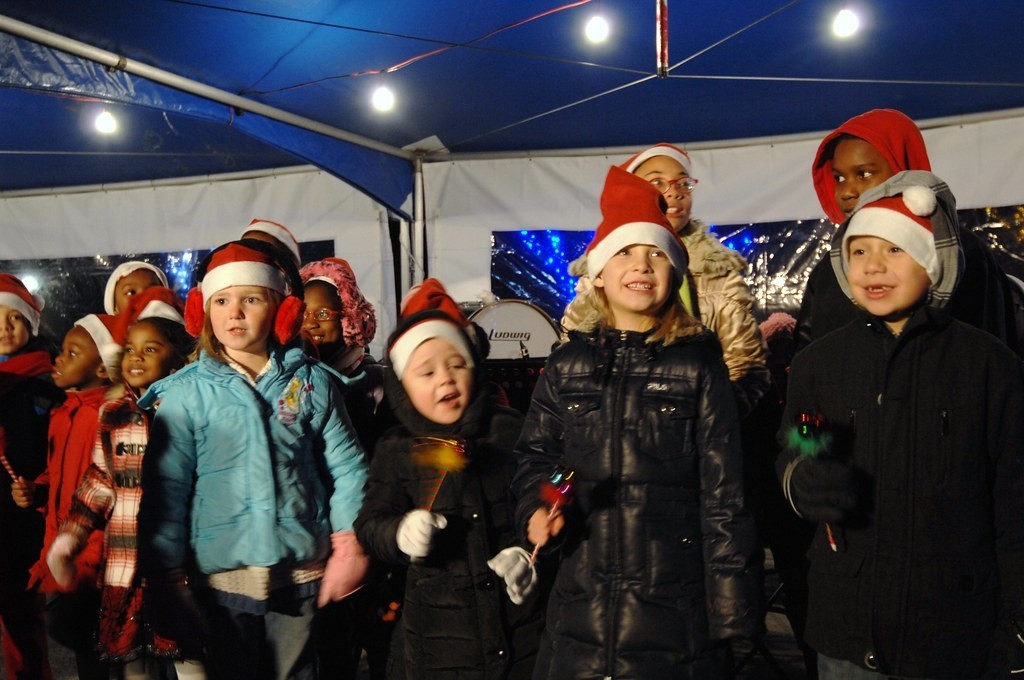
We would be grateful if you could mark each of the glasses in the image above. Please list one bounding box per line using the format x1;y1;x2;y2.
649;177;698;194
303;308;342;322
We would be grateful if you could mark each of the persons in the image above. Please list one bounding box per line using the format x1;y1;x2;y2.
0;110;1024;680
352;309;551;680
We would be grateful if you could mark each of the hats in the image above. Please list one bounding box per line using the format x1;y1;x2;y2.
201;243;291;313
124;286;185;325
0;273;44;336
241;219;300;260
586;165;690;284
841;186;937;285
104;261;169;315
617;142;692;176
758;312;796;342
387;277;473;382
303;258;356;288
74;313;129;382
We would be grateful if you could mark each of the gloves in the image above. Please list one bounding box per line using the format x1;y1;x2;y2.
318;529;368;607
987;619;1024;680
47;534;83;586
396;509;447;556
487;546;537;606
773;443;849;520
144;579;190;629
